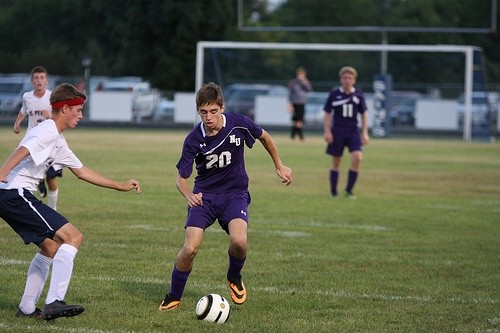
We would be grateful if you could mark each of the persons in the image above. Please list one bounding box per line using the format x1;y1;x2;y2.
322;66;369;199
287;68;313;145
0;83;141;321
158;82;291;311
13;65;63;211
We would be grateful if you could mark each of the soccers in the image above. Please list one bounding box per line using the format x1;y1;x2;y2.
195;294;230;324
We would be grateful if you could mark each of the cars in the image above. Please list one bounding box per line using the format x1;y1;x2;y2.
93;80;161;125
0;73;66;125
386;89;422;127
454;90;500;139
223;82;290;121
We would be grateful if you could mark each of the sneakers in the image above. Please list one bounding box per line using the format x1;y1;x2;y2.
158;292;180;312
15;307;44;319
42;300;84;320
227;277;247;305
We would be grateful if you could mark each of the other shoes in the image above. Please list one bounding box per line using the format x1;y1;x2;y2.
343;190;355;198
